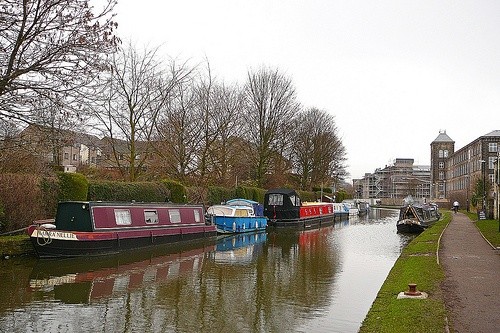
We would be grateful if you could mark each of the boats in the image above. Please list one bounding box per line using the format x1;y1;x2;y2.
357;201;370;218
206;197;268;235
395;200;441;231
263;188;335;224
29;200;219;258
333;202;360;216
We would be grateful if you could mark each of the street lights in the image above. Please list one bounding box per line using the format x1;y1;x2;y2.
481;160;486;215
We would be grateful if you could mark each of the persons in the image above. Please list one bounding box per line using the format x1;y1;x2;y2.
452;199;460;212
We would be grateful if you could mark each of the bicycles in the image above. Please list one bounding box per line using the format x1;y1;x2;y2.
454;207;458;214
